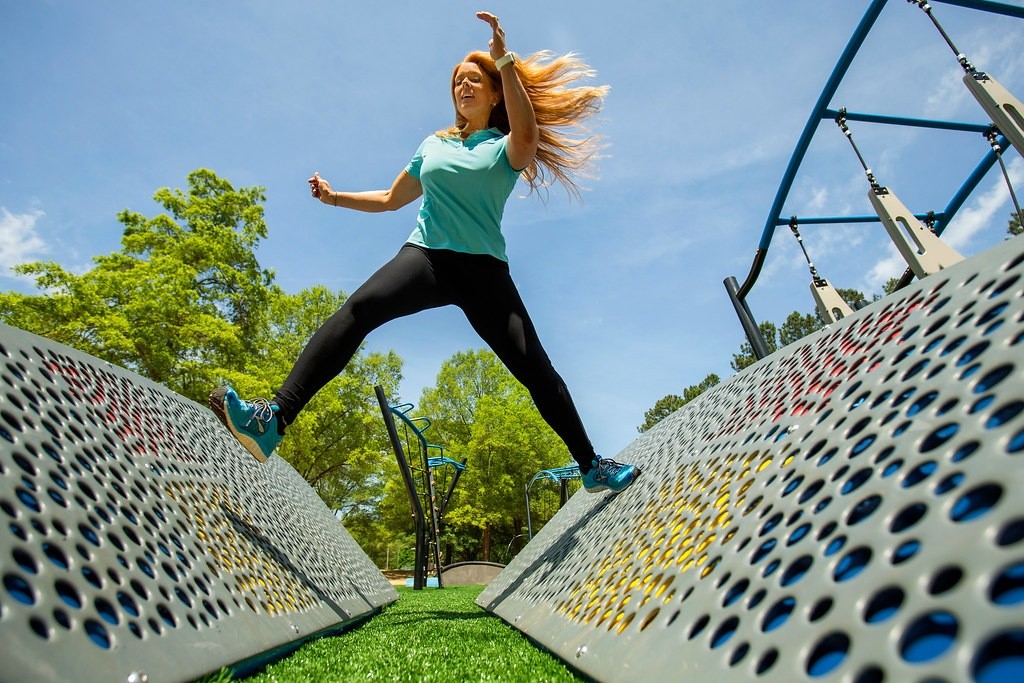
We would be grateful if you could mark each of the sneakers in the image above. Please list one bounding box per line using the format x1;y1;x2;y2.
209;385;286;463
579;455;638;493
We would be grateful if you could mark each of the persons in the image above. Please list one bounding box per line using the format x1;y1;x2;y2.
207;10;641;493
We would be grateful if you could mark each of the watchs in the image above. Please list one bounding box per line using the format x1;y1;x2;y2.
495;52;515;72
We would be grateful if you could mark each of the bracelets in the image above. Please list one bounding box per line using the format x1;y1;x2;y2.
335;191;338;207
